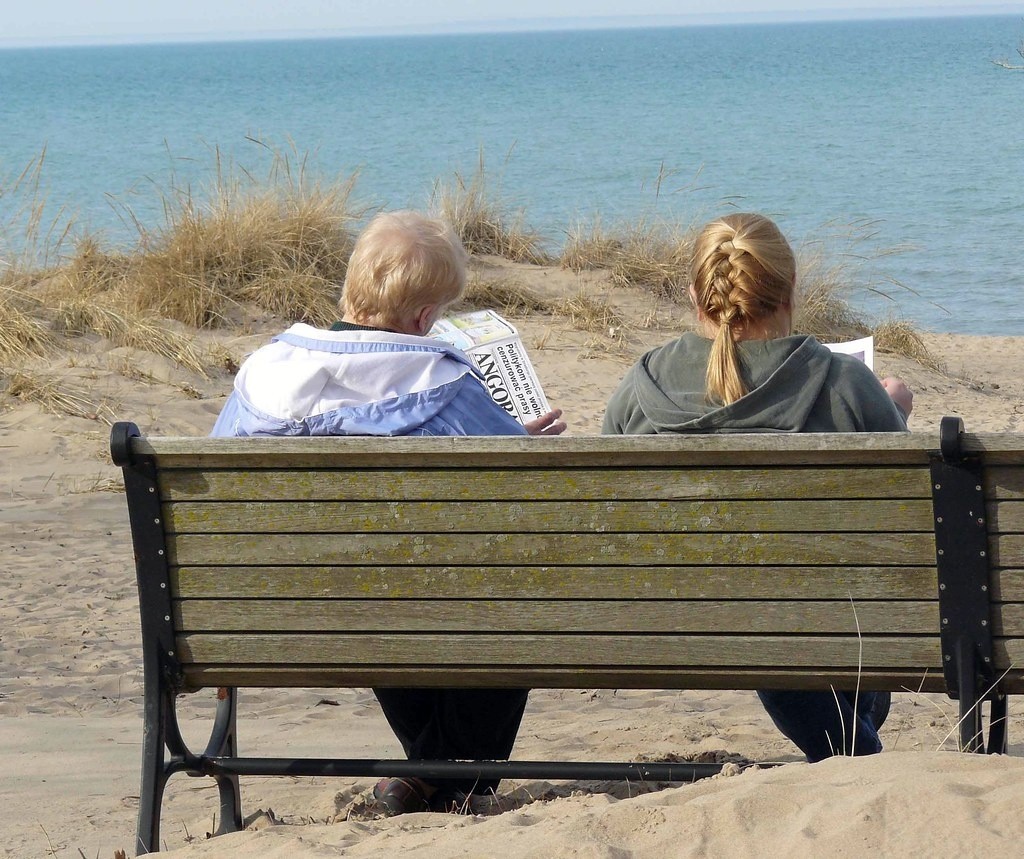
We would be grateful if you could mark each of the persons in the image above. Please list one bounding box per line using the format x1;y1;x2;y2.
602;211;914;763
209;210;567;816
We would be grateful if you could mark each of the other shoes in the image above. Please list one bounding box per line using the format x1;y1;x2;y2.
374;776;426;817
871;690;891;730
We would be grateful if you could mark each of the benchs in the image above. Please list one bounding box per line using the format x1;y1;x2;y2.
110;422;1024;855
